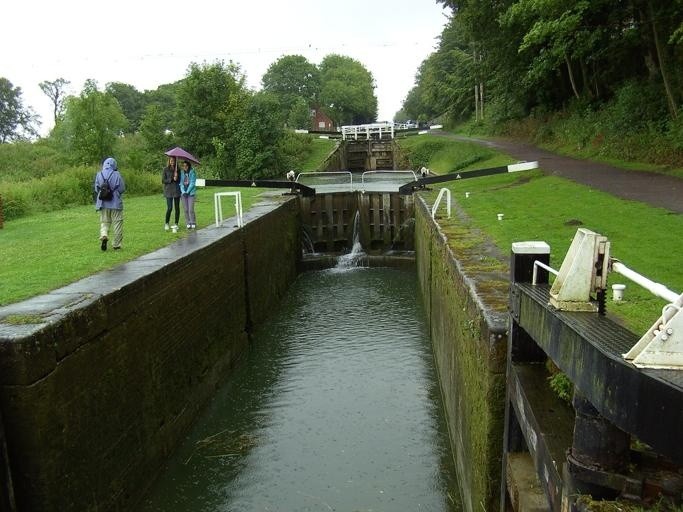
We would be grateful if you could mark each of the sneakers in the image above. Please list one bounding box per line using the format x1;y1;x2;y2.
164;224;179;233
187;224;196;229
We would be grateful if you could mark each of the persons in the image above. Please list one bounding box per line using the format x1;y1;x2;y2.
178;160;197;230
94;158;125;251
161;157;181;231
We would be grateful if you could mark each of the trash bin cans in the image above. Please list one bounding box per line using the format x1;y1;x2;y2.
510;241;551;283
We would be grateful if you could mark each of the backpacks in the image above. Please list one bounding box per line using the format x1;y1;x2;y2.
99;170;114;201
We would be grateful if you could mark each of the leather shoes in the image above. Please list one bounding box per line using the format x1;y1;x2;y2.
113;246;120;249
101;236;108;251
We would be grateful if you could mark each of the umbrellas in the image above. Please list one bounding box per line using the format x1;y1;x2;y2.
163;147;199;177
